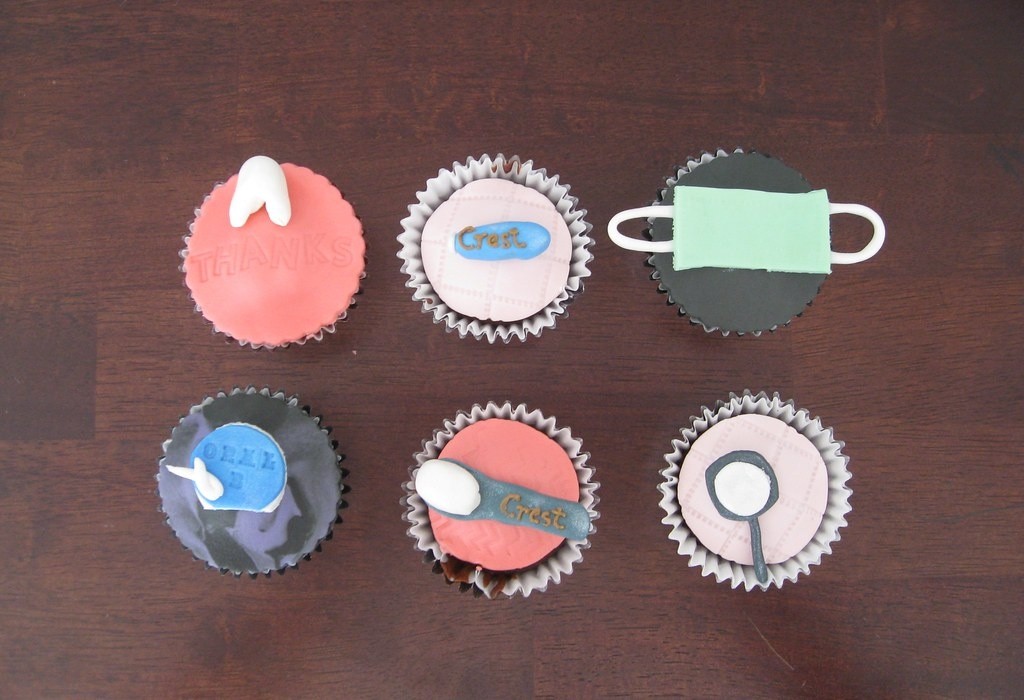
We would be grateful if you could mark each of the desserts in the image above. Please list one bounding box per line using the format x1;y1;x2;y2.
398;400;602;600
658;387;853;591
176;155;371;352
608;147;886;337
396;154;596;344
154;384;353;577
